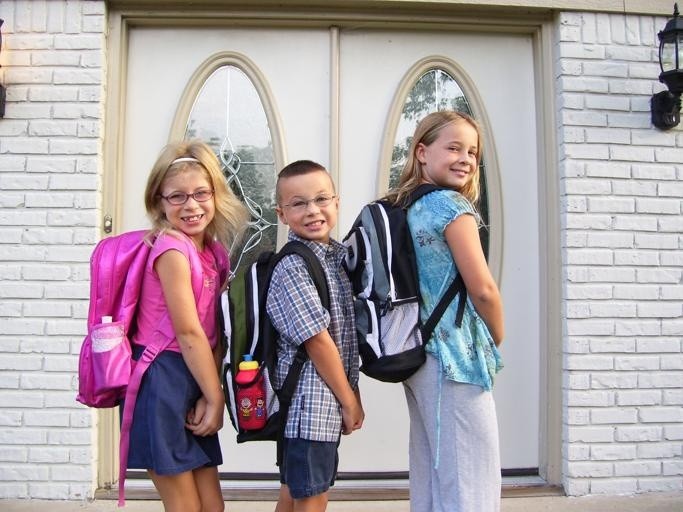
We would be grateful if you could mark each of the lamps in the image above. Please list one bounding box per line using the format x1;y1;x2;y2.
648;1;682;129
0;16;7;119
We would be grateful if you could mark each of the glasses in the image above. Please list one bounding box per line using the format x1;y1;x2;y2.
277;193;338;211
156;187;217;206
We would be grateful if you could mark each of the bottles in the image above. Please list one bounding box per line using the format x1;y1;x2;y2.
232;355;269;431
90;313;125;354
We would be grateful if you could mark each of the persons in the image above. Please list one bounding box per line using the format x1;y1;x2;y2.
403;111;506;512
267;160;364;512
119;139;250;512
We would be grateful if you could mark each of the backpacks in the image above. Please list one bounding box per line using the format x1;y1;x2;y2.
340;182;477;384
74;224;204;410
214;238;332;445
